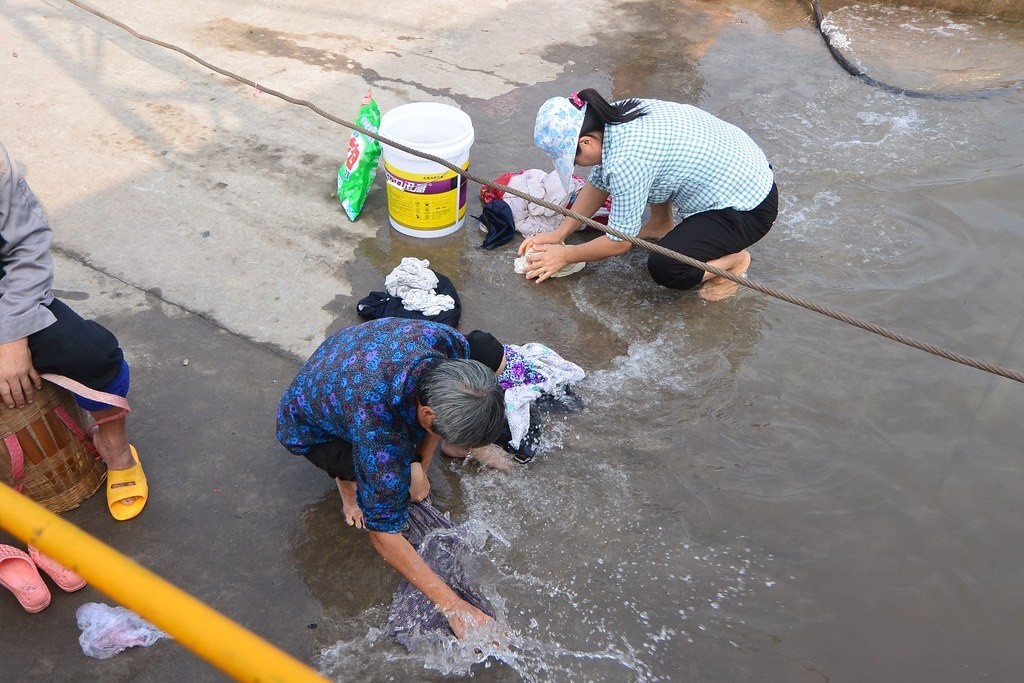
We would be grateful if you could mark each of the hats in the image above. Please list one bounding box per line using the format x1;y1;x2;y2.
533;96;588;195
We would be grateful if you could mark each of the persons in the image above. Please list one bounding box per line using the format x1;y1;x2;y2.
0;139;150;519
275;316;541;645
518;88;782;301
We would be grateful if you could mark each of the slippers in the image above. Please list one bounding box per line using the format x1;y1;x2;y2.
107;443;149;521
0;542;88;614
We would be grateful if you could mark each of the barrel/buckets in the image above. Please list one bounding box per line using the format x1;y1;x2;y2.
377;101;476;239
